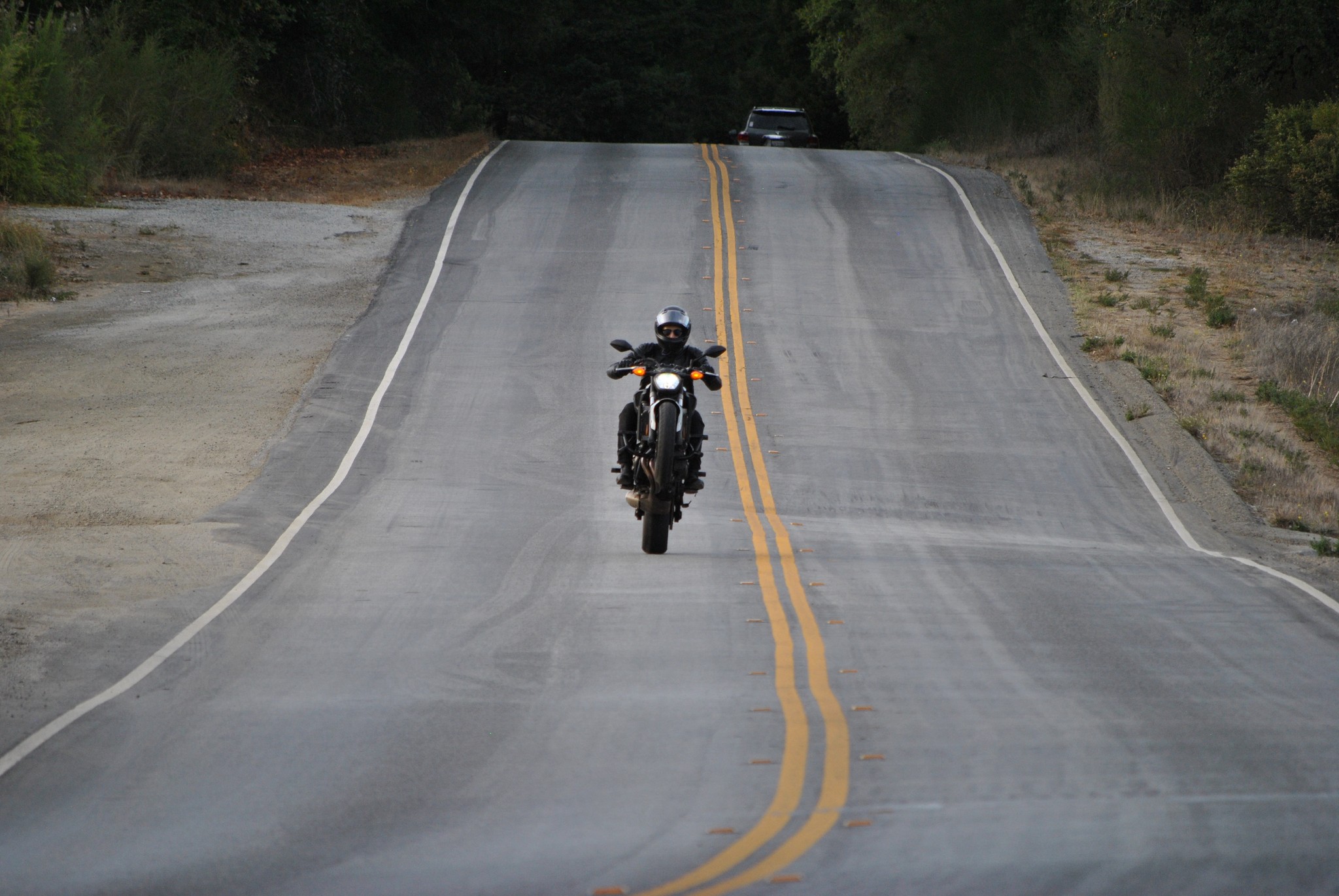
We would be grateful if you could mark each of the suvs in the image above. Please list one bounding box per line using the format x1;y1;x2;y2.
736;106;820;149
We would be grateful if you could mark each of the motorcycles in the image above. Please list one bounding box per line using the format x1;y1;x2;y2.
607;338;727;554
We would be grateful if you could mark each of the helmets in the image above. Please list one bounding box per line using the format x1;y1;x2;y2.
655;306;691;350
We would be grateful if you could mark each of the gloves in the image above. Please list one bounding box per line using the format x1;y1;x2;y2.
701;364;714;373
619;359;632;368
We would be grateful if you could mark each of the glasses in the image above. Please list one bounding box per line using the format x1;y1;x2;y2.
661;329;683;336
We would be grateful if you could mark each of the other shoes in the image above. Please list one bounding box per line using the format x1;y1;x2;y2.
616;462;634;484
681;468;704;490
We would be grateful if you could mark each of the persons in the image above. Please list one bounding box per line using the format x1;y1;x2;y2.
606;306;722;495
